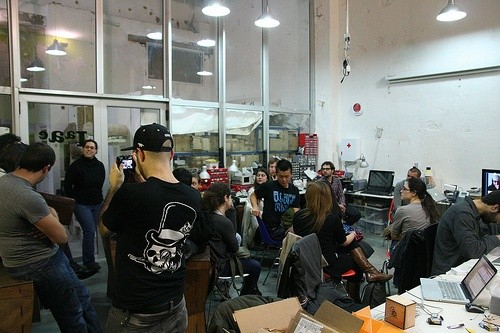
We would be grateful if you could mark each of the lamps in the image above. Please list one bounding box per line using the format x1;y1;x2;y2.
45;39;67;56
26;58;45;72
255;0;280;29
435;0;467;22
344;156;367;172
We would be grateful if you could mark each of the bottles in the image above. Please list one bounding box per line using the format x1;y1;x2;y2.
425;167;434;188
199;166;210;179
227;160;238;172
303;176;308;189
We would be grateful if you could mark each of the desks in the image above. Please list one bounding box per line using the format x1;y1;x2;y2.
0;269;34;333
184;246;211;333
370;245;500;333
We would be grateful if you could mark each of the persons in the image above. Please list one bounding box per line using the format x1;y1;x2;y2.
172;158;500;295
65;139;106;270
122;160;132;168
0;133;99;333
99;124;203;333
487;175;500;191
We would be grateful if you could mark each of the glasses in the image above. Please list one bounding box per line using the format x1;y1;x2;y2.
321;168;331;171
402;186;414;193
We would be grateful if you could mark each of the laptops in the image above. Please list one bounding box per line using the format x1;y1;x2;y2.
361;170;395;195
420;253;496;304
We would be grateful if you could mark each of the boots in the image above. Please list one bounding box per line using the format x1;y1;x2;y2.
352;247;393;283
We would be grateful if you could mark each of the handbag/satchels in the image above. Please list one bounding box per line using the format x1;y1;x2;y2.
236;247;250;258
38;192;75;226
299;280;354;315
344;205;361;226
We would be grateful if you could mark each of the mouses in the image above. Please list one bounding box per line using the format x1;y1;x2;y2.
466;304;484;313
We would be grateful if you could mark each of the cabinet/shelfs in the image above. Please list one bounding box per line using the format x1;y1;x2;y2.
346;192;453;225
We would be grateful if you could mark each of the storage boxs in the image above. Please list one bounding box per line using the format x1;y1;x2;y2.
384;295;416;330
231;296;365;333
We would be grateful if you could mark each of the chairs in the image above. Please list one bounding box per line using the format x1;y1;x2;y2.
217;273;250;305
382;222;438;295
255;216;282;285
277;233;357;279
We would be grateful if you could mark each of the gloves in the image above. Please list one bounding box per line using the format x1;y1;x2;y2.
235;233;242;246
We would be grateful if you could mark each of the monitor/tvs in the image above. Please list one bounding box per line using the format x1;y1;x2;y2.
481;169;500;198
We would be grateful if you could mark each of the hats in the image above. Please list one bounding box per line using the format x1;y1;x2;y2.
120;123;173;152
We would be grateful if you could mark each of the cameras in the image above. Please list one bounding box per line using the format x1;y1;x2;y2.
117;155;133;170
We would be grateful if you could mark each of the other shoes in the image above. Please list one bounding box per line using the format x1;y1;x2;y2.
83;256;101;271
69;261;96;280
